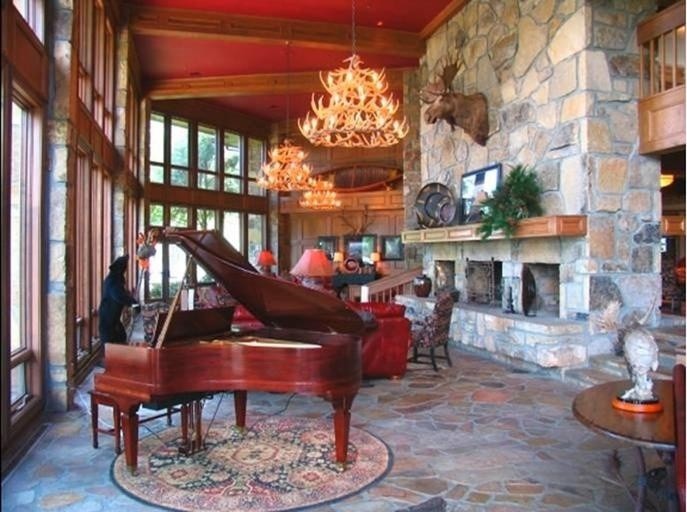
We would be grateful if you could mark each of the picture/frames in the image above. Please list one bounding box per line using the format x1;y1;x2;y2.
458;163;502;226
318;234;404;268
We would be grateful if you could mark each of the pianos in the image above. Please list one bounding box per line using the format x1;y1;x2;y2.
87;226;379;473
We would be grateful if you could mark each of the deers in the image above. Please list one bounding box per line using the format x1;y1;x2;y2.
414;50;489;147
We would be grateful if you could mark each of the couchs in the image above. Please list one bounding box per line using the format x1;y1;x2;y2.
229;301;411;381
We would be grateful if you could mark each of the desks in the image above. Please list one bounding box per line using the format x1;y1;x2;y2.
572;379;677;512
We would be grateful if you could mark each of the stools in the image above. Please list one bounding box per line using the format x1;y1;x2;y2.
85;388;195;454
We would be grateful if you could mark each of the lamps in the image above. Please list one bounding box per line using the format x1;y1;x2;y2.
289;248;338;290
254;250;278;274
256;1;410;211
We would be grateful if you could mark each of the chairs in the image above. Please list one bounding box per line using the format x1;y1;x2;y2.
407;293;454;371
661;252;682;312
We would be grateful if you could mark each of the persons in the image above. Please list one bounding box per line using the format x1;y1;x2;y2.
98;253;140;345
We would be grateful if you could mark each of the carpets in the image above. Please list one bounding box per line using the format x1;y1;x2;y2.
111;415;394;512
